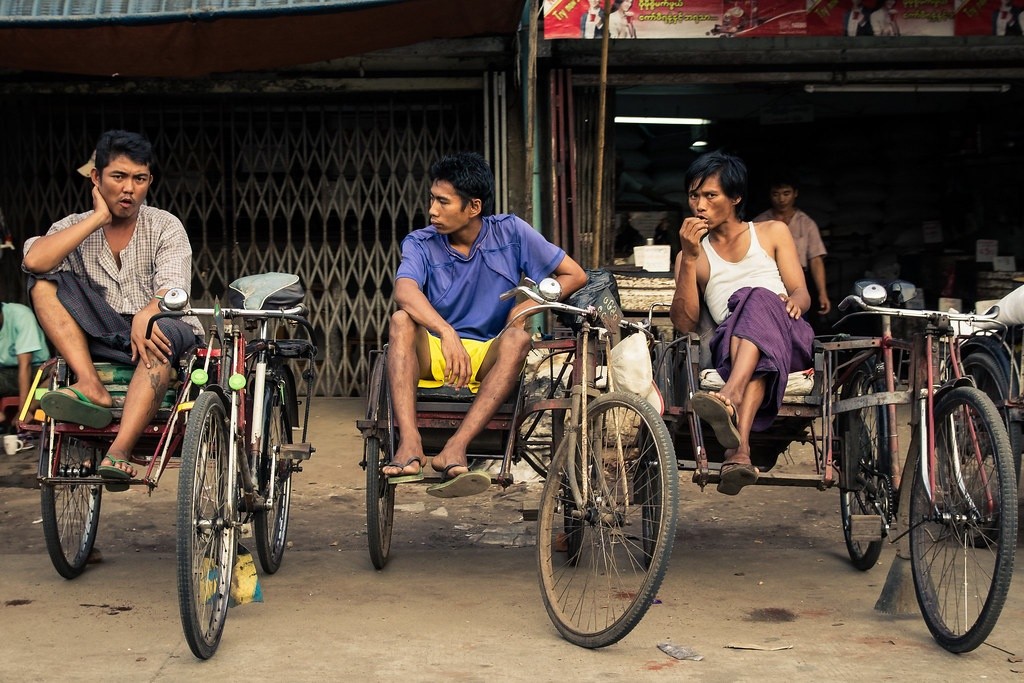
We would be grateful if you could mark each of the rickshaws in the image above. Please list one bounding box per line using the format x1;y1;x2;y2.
354;277;681;650
612;268;1024;655
17;269;319;660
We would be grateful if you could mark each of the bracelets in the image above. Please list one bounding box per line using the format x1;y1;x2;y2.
155;295;163;300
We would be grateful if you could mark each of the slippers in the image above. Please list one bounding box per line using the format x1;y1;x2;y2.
691;392;741;448
40;387;113;429
717;462;758;495
387;456;424;483
98;454;137;492
425;464;491;498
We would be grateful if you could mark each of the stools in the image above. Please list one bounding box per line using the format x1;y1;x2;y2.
0;396;19;414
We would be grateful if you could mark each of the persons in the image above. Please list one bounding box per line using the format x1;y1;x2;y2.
0;300;51;434
21;131;205;491
753;177;831;315
669;150;815;496
381;152;589;499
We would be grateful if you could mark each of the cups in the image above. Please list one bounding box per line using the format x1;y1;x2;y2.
889;10;897;21
1001;10;1008;19
625;13;632;23
589;10;596;21
852;11;862;20
3;434;24;455
647;239;653;246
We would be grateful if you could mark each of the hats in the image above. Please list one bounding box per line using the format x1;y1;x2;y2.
609;330;664;417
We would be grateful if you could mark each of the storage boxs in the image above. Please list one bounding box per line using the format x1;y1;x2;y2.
634;245;670;272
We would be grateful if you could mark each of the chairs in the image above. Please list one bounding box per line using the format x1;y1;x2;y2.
673;295;823;417
19;350;220;435
378;387;520;429
